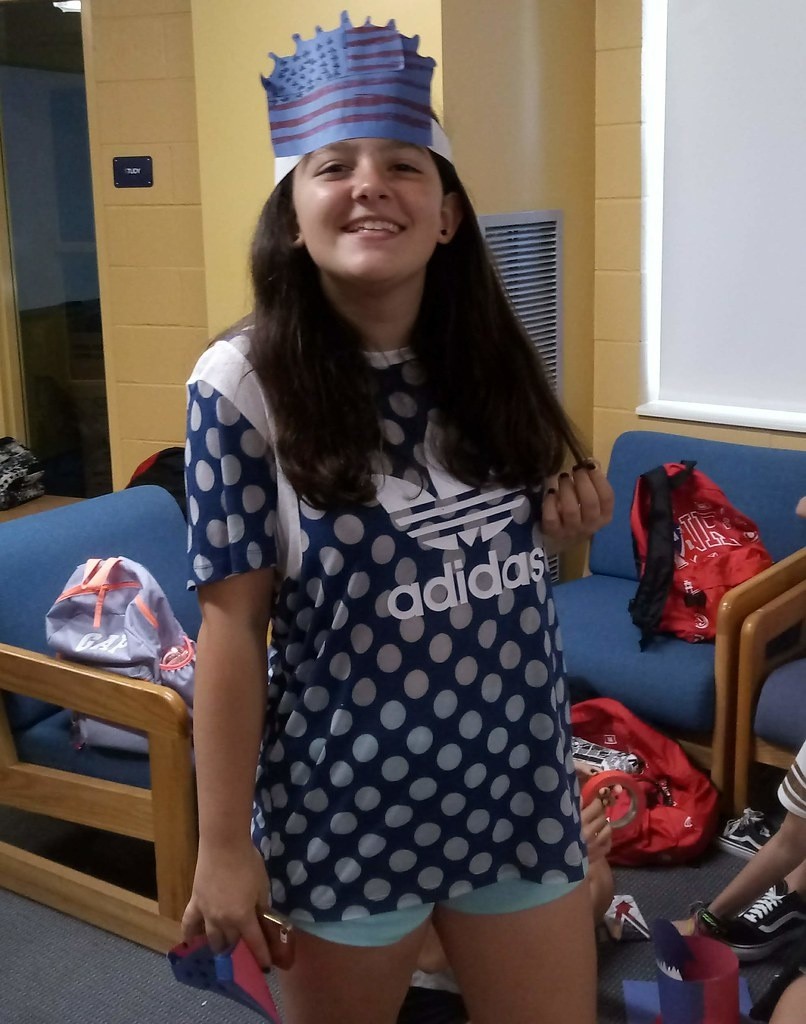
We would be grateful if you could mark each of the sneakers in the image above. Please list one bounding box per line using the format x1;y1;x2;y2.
713;807;779;862
711;879;806;964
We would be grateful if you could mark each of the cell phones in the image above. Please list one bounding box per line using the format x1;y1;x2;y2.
257;908;295;973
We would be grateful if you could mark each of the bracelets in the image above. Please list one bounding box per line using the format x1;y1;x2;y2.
688;900;721;935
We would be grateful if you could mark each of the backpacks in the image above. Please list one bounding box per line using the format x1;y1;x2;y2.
626;454;776;650
0;436;50;514
563;691;721;871
44;557;205;757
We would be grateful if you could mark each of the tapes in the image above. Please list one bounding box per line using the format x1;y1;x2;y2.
581;770;646;838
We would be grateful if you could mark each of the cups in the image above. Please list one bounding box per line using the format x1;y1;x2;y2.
655;936;740;1024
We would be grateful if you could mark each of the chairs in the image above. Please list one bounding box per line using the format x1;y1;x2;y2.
550;431;806;803
733;583;806;815
1;485;240;956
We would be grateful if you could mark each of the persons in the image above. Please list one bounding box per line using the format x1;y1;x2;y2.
395;763;615;1023
181;9;615;1024
673;735;806;1024
